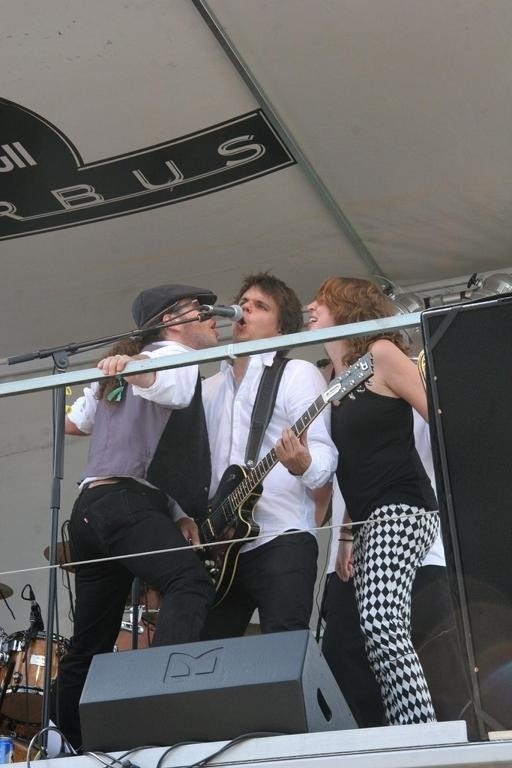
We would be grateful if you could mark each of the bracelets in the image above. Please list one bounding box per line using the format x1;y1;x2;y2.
339;526;354;536
338;538;354;543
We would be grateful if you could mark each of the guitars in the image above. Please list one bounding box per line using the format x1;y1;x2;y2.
190;352;375;609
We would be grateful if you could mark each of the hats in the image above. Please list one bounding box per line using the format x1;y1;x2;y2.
132;284;217;329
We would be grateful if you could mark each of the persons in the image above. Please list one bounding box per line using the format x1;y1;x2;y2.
159;269;341;643
305;274;443;727
43;283;220;750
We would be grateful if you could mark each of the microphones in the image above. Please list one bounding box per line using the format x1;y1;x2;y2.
202;303;244;322
30;589;45;631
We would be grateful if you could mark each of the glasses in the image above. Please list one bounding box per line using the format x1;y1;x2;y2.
170;299;200;312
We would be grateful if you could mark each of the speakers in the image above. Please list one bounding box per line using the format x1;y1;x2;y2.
77;628;360;752
418;292;512;740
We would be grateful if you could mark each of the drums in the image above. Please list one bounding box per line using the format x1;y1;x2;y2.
0;631;69;726
10;720;47;764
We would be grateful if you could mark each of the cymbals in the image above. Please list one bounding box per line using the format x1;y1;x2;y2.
0;582;13;600
44;538;75;574
